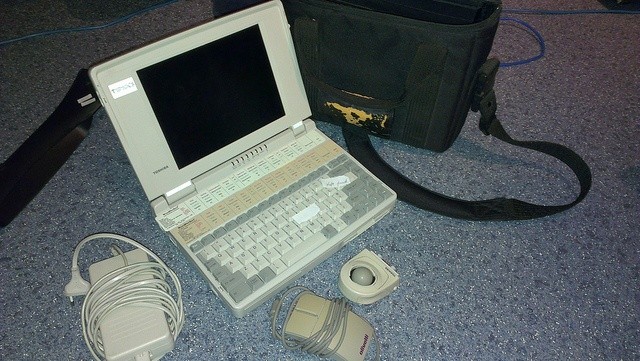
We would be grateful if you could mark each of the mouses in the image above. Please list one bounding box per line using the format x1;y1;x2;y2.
269;286;380;361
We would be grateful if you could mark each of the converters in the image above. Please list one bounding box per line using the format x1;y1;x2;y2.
64;232;184;361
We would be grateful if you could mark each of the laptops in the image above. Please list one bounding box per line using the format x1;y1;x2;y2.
87;0;398;318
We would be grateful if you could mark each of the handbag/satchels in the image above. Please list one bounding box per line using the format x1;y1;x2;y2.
210;2;592;220
